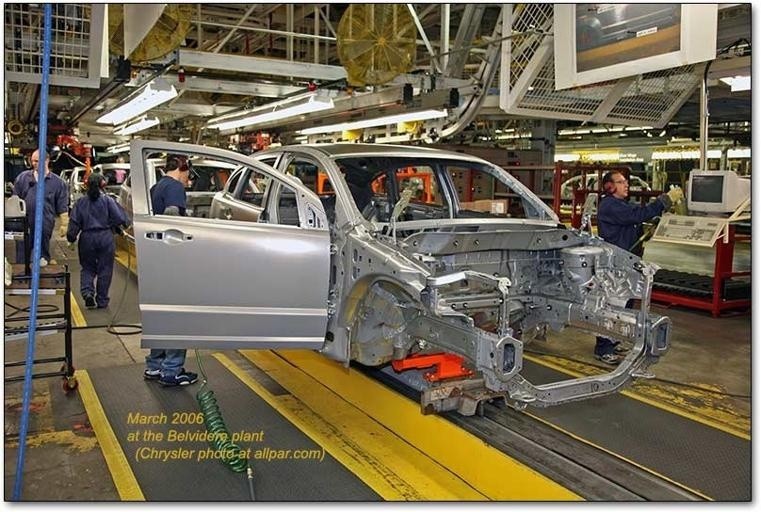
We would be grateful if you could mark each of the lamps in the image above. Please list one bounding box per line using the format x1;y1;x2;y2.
205;94;452;137
99;76;179;138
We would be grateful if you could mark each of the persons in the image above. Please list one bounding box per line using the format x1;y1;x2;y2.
594;169;684;365
408;167;419;196
66;172;130;308
12;148;67;264
113;157;128;184
143;152;199;387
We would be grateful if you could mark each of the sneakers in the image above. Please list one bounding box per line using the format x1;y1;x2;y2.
85;295;111;308
594;345;632;366
143;366;162;380
159;367;200;386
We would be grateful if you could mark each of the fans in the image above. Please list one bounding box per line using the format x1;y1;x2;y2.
107;2;194;64
337;3;419;86
